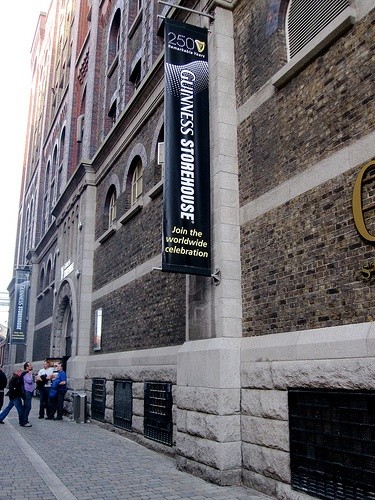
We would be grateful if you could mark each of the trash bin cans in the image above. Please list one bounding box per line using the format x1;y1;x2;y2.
72;392;87;423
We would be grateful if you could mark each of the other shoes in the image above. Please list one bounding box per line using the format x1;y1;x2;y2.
24;422;32;427
0;420;5;424
38;416;64;420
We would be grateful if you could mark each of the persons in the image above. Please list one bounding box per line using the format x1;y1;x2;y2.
0;368;32;427
19;362;34;427
0;365;8;410
36;358;66;420
